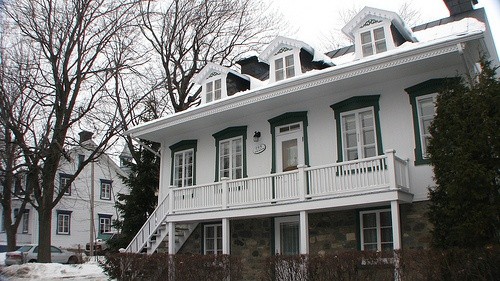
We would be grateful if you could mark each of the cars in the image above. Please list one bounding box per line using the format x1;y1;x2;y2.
5;244;81;266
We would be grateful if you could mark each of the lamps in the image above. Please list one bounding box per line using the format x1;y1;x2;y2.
253;131;261;142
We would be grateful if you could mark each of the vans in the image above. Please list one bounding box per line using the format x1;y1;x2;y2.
84;231;122;255
